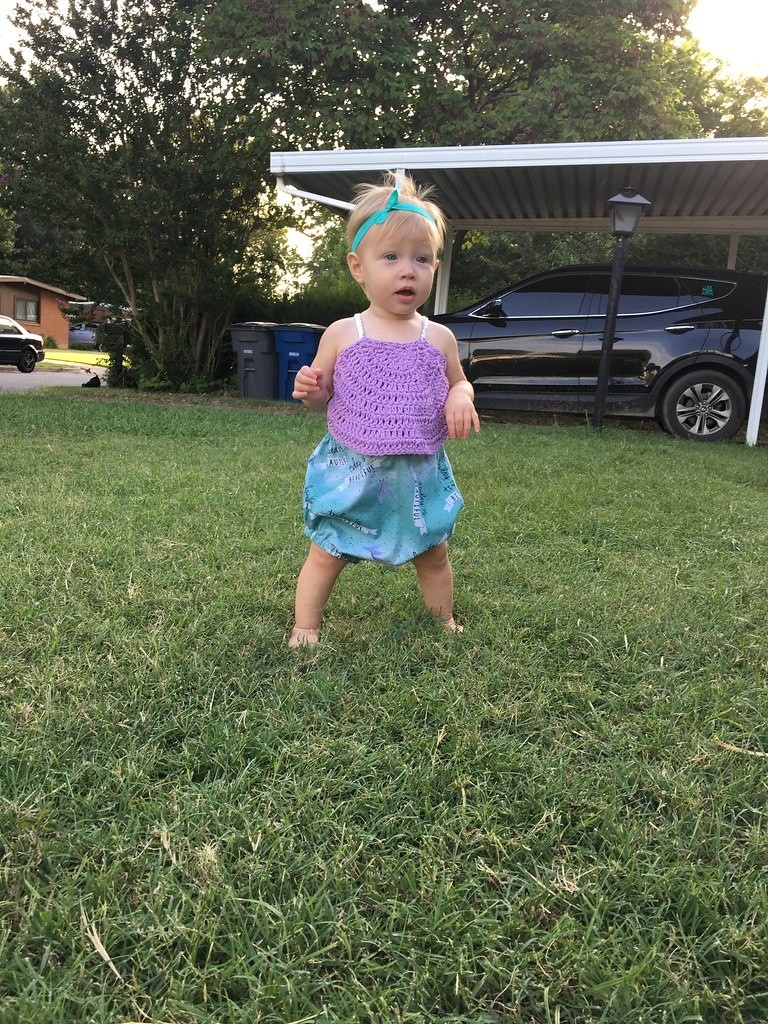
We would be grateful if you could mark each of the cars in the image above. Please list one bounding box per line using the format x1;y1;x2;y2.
0;314;46;373
69;320;107;353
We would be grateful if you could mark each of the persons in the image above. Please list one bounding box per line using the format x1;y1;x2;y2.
288;171;480;647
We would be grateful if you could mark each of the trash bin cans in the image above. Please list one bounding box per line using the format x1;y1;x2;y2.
226;320;278;402
274;323;328;404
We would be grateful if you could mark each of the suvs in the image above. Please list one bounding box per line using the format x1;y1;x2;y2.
427;258;768;444
